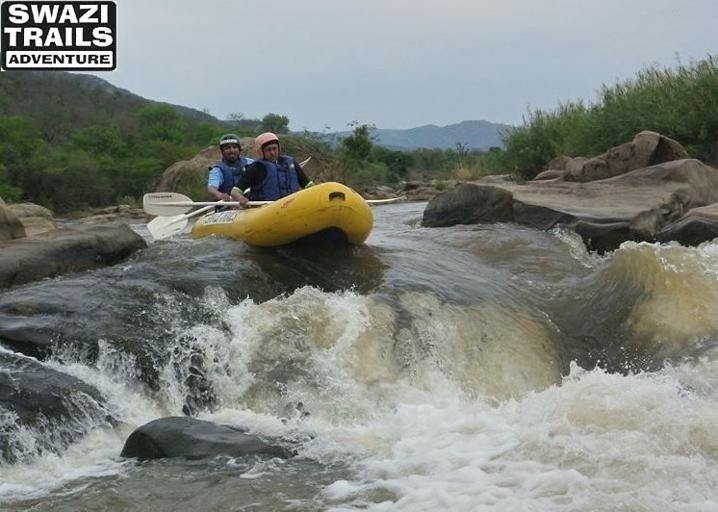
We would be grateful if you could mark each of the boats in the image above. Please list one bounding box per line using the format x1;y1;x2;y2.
191;181;372;248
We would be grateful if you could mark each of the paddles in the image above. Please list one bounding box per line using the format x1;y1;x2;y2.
147;156;313;240
143;192;403;216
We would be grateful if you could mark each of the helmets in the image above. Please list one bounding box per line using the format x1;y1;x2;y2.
219;134;242;151
254;133;279;160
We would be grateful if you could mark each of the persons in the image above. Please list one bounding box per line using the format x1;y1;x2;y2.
230;132;316;208
206;133;255;210
